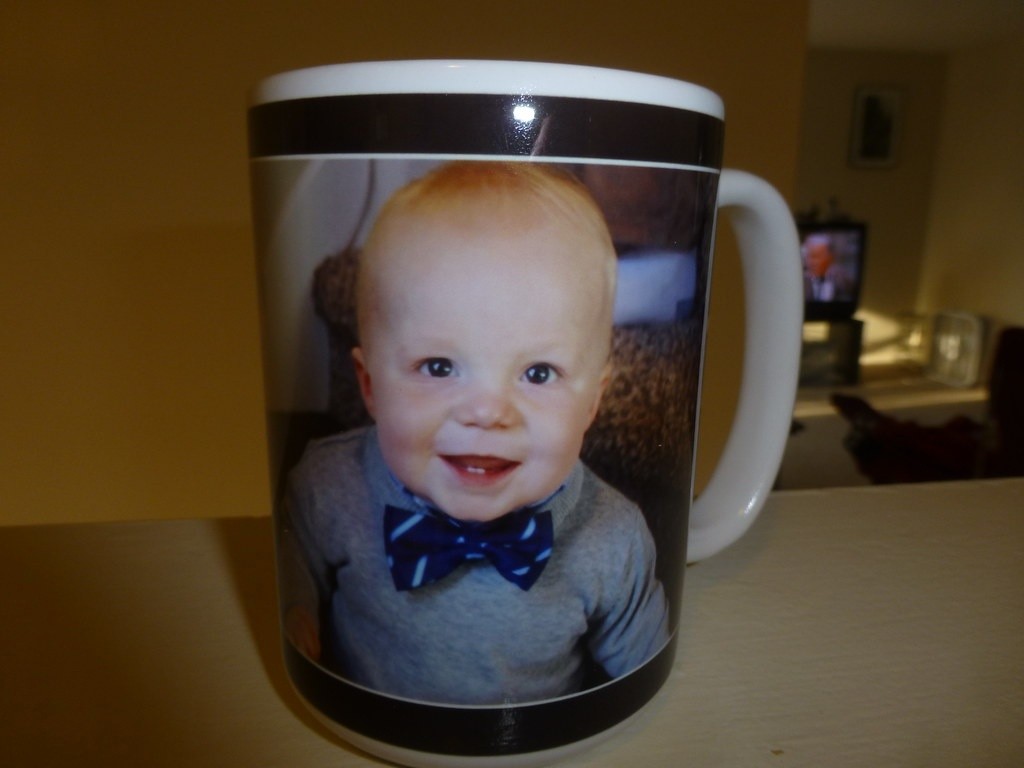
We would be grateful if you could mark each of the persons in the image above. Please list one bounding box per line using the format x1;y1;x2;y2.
833;327;1024;481
285;162;672;708
800;234;850;302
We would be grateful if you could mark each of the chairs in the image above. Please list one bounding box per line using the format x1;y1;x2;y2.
830;326;1024;486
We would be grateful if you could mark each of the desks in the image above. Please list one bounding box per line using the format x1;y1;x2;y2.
0;478;1024;768
776;378;988;488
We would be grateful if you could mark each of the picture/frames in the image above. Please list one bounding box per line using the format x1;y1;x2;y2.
848;81;905;169
926;312;984;384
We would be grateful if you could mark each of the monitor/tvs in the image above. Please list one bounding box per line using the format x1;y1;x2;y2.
796;218;867;322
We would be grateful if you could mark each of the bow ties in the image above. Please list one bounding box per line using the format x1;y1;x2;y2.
383;503;557;593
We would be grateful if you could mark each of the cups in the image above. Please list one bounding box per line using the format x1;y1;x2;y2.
245;59;805;768
